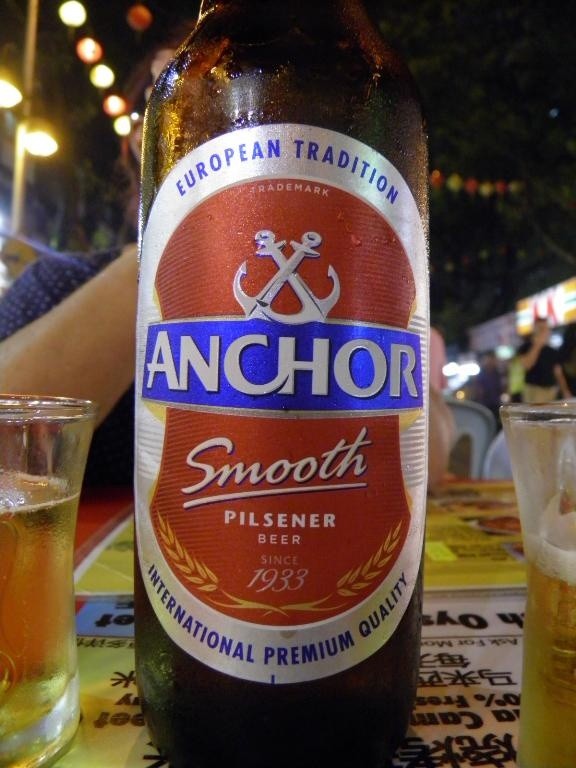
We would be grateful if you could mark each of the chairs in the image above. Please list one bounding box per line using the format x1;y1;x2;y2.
443;401;498;479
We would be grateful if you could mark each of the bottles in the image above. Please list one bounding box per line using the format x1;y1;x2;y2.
130;2;433;767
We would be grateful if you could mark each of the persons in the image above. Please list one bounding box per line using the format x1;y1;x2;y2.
0;28;452;538
453;306;576;433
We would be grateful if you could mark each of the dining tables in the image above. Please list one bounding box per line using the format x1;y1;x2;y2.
43;476;525;768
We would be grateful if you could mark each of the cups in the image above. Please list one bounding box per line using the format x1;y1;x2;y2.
1;389;103;768
498;388;576;767
